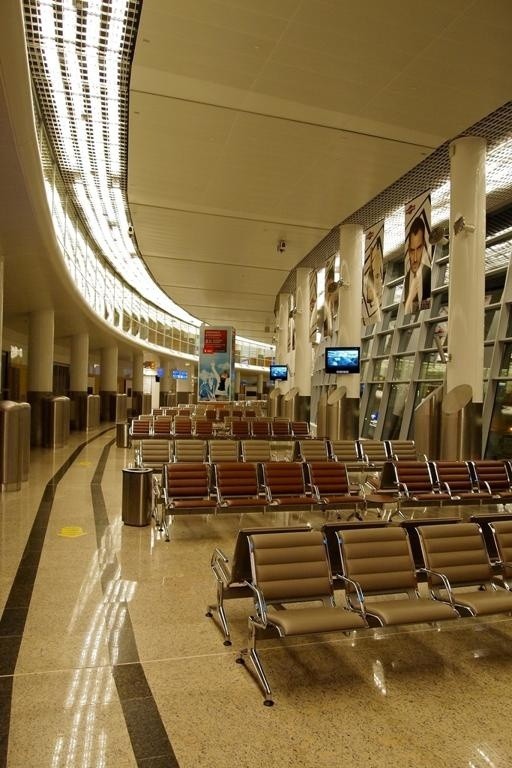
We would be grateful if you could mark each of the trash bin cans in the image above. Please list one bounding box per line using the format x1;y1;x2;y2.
116;423;131;448
121;468;154;527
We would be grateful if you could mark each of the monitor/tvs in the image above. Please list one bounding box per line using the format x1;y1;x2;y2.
269;365;288;381
324;346;361;374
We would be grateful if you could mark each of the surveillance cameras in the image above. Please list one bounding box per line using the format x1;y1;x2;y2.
278;242;287;251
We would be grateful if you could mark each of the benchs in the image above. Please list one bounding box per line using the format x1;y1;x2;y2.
237;517;512;707
206;511;512;649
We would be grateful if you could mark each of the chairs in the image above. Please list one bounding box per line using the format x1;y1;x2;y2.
131;392;512;541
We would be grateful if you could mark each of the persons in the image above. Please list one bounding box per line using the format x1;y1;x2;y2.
210;360;230;400
405;217;431;301
325;270;334;339
